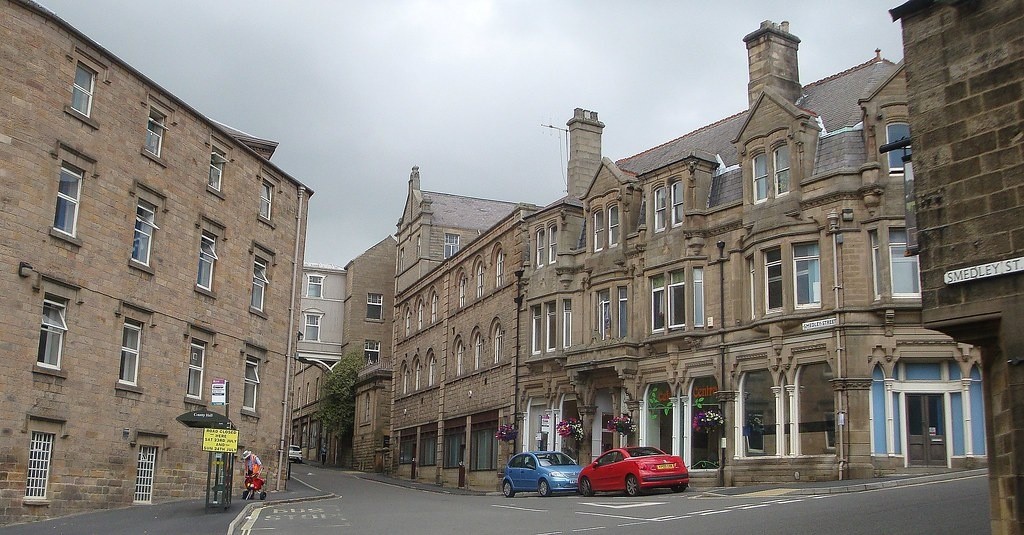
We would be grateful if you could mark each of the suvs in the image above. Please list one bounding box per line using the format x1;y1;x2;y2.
289;445;303;462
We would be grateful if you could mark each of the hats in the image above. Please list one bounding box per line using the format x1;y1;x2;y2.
243;450;251;459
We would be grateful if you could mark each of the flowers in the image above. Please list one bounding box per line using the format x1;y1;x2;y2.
495;423;518;441
557;418;584;440
692;409;726;433
607;416;637;437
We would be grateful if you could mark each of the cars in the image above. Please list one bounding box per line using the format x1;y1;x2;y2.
501;450;584;497
578;446;690;496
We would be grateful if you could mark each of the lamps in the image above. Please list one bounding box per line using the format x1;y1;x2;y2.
18;261;33;278
842;208;854;221
298;331;304;340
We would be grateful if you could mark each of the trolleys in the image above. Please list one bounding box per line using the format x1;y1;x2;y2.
243;468;267;500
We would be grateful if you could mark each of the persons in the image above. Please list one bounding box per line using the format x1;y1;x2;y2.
242;450;263;499
319;445;328;466
